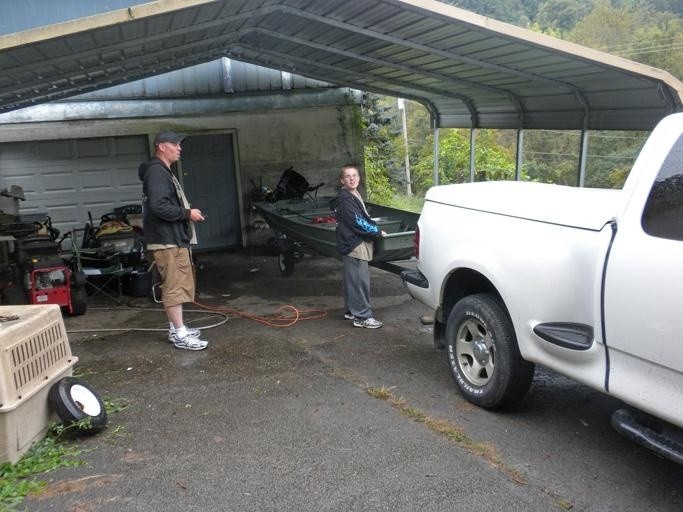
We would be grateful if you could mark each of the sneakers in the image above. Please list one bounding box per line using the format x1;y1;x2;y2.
168;328;208;351
344;312;384;329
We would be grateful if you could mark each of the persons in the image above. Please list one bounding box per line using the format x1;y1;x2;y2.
335;164;386;329
138;131;208;350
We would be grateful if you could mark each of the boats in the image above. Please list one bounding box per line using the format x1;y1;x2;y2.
250;196;421;262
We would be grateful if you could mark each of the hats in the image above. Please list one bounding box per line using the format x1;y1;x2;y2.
154;132;186;144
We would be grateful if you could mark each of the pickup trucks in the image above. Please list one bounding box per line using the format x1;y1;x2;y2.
395;111;682;466
